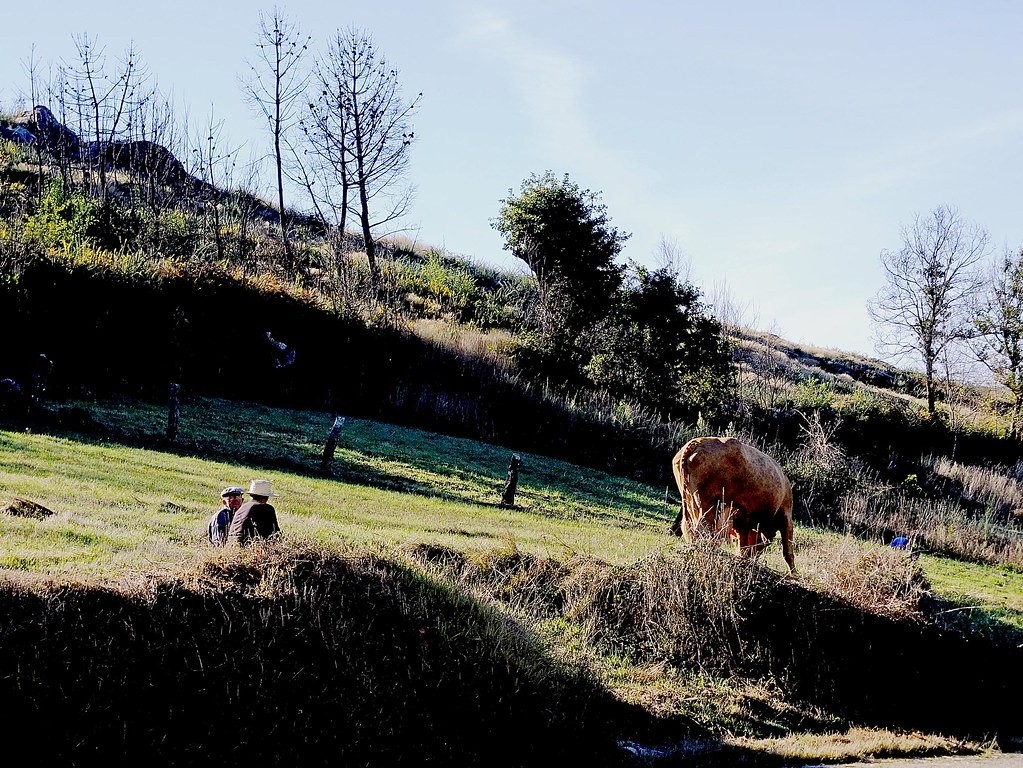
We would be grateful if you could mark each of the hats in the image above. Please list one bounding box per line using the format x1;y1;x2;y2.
246;480;279;496
221;487;243;497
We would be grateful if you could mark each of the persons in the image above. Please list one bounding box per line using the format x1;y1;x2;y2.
208;487;245;547
226;478;282;548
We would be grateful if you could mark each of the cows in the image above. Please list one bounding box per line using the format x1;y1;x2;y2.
673;436;797;575
